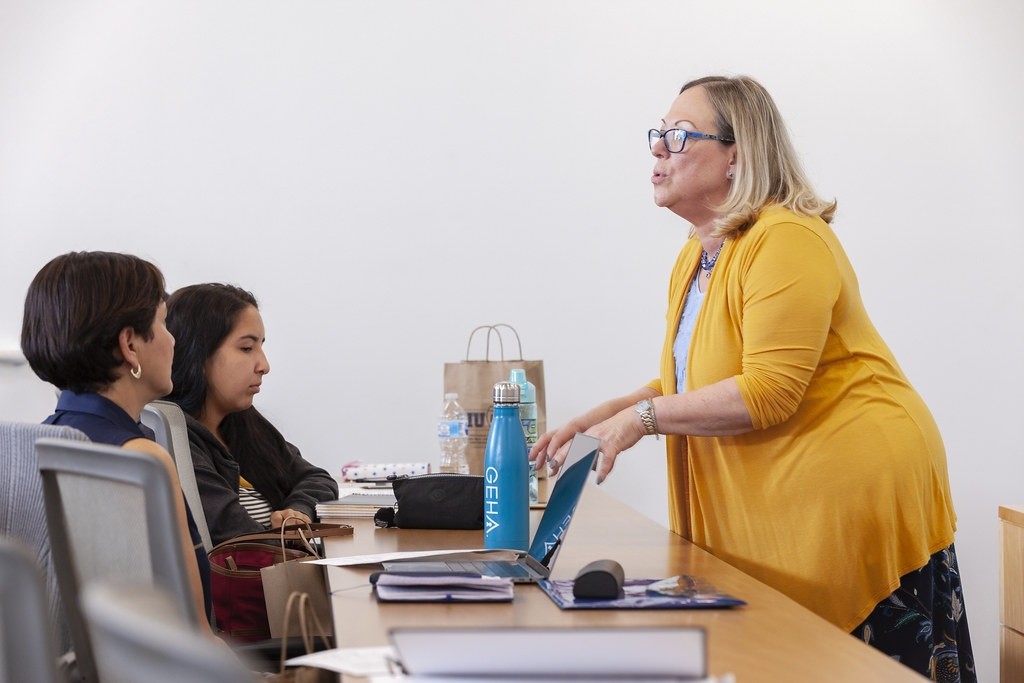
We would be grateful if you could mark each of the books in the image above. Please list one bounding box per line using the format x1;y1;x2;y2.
315;492;398;518
376;574;515;602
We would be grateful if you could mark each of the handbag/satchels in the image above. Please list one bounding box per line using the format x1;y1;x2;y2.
265;592;333;683
207;523;353;643
260;517;334;638
373;473;494;530
443;324;551;504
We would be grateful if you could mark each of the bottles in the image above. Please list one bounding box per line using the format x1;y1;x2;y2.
484;381;530;553
509;369;538;504
439;392;471;474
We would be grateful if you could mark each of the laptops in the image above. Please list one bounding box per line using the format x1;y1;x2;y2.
383;431;602;584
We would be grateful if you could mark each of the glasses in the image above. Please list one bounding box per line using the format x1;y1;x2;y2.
648;128;736;153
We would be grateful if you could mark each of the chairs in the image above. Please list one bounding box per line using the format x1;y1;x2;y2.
0;399;263;683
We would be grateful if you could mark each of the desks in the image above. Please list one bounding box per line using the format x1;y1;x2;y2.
316;466;932;683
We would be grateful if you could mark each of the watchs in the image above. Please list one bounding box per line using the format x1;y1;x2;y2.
636;400;655;435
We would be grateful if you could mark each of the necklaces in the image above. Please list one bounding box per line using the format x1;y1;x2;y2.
700;237;725;278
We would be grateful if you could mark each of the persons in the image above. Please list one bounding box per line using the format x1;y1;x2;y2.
20;251;340;683
530;75;978;683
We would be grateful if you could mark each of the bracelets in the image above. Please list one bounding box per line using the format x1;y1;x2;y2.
648;396;659;436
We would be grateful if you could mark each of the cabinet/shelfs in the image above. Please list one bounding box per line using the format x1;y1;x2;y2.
999;504;1024;683
